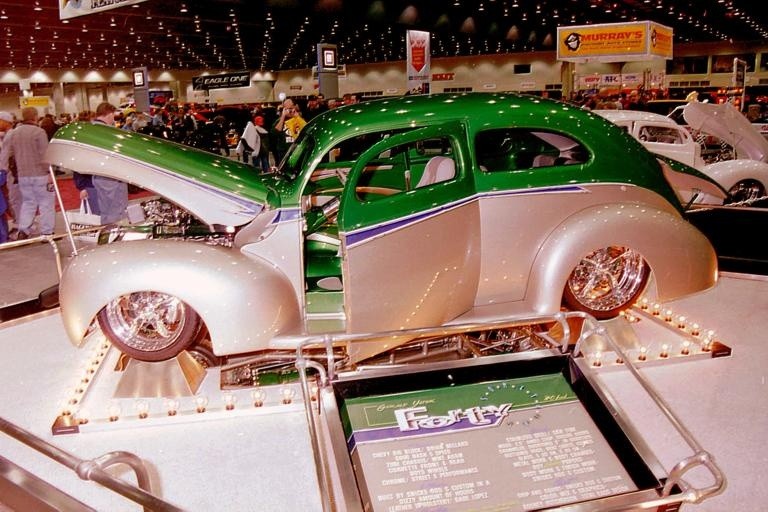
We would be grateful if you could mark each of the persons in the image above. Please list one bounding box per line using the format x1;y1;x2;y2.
0;74;362;242
560;86;652;112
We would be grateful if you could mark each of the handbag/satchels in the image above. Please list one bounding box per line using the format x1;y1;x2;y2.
65;210;101;241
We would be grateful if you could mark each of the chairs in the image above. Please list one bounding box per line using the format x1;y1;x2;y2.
416;156;456;189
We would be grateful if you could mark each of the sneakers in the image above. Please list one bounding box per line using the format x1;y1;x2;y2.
17;232;31;239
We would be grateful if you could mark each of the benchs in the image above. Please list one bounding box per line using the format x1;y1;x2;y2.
532;154;579;169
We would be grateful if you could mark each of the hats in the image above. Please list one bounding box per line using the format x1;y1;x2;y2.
0;112;13;122
